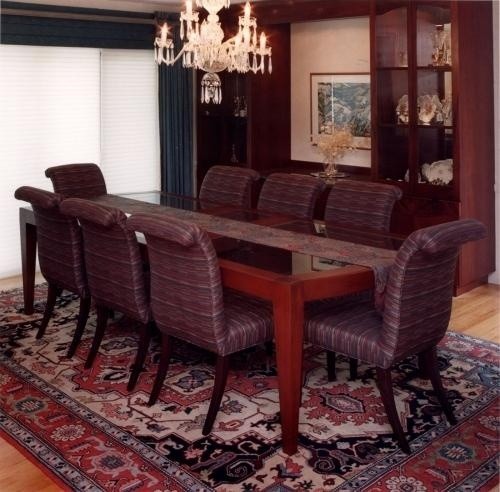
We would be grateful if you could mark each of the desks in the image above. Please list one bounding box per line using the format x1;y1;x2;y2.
14;187;417;454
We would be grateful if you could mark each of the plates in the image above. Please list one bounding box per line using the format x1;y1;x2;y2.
394;94;409;123
416;95;437;124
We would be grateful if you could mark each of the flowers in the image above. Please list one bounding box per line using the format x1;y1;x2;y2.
305;121;362;162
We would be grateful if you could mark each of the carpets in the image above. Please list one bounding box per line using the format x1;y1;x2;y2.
0;276;500;492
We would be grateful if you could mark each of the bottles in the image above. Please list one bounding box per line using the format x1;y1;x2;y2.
431;23;448;66
233;96;248;119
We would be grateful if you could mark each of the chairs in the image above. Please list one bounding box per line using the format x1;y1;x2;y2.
305;215;490;454
55;192;156;394
258;165;326;224
44;162;111;200
127;209;276;435
311;178;406;384
192;163;257;214
14;182;86;362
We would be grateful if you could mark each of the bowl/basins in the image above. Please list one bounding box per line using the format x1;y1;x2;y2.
422;158;454;185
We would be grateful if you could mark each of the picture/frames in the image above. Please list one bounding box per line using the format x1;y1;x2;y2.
308;71;374;152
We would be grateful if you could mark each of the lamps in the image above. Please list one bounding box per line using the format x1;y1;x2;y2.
146;0;273;109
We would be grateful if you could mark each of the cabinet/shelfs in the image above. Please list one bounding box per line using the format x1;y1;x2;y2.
196;24;289;192
255;176;461;297
369;0;494;205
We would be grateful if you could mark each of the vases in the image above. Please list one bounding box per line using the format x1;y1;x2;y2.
321;156;338;178
